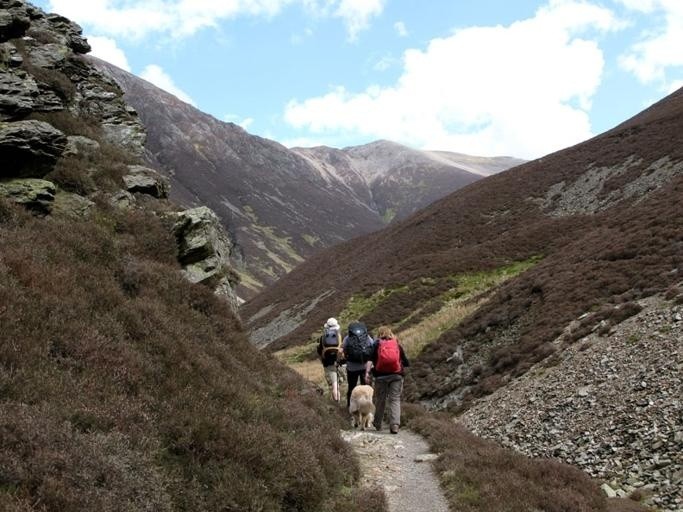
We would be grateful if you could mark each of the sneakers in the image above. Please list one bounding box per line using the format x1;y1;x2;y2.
390;424;399;434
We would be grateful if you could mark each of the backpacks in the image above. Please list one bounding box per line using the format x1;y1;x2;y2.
373;337;402;374
343;322;373;363
319;329;341;363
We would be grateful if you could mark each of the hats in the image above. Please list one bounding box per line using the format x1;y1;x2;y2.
323;317;340;330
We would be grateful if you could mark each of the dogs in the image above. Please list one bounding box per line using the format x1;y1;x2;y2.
349;385;375;432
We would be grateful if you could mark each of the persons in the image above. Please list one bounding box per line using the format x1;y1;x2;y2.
316;317;345;403
338;320;374;408
365;326;410;434
